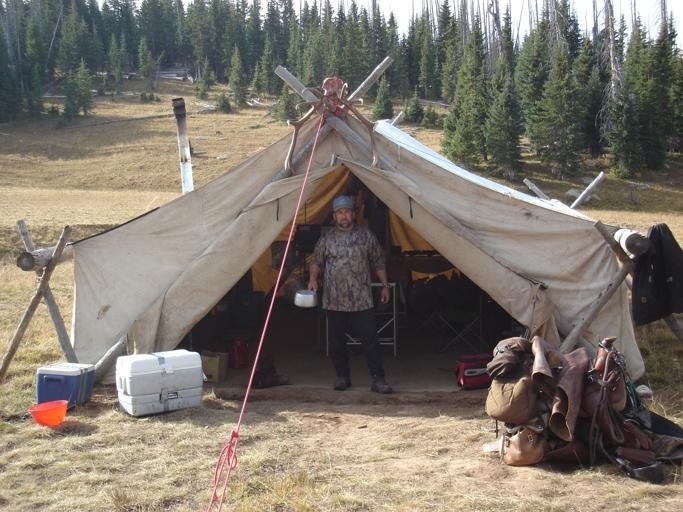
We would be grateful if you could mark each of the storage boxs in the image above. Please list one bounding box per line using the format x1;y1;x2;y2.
201;350;229;383
116;348;208;418
35;363;95;413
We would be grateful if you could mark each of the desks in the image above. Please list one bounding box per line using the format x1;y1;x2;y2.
326;282;398;357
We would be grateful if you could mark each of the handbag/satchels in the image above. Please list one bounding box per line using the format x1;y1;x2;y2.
249;351;288;386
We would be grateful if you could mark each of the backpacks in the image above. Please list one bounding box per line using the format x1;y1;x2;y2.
485;335;633;466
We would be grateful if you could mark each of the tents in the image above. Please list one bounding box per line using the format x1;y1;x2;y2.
68;78;644;402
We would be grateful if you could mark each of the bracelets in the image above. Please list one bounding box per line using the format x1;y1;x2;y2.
381;282;390;288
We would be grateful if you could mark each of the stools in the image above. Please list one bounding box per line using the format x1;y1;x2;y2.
439;303;480;352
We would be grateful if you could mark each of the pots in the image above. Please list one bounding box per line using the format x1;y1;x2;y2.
294;290;318;308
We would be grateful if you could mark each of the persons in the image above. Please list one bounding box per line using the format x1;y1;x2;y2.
307;196;394;393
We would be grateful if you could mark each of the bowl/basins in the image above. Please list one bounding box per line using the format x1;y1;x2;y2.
28;400;68;426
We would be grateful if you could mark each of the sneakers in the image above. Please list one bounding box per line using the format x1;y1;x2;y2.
334;376;352;390
371;380;391;393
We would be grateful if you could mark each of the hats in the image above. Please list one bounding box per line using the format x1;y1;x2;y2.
333;196;354;213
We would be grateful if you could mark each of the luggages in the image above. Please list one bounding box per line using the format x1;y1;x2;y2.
455;352;495;389
